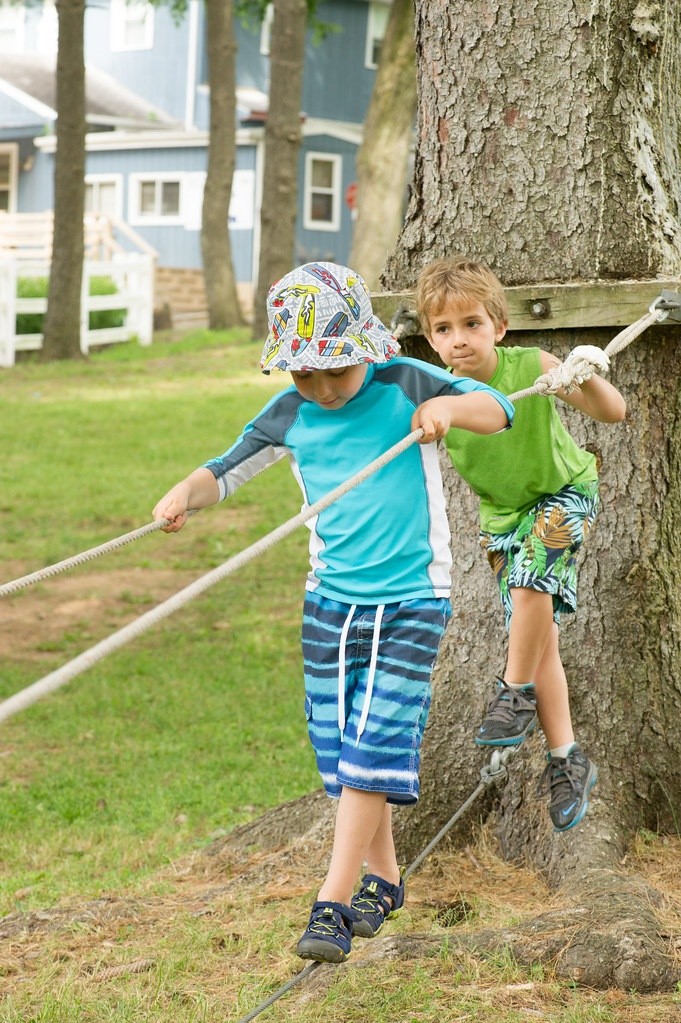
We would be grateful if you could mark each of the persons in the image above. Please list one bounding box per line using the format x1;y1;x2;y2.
415;256;629;832
151;260;516;966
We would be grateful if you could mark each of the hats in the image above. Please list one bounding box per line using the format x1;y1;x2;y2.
260;261;401;374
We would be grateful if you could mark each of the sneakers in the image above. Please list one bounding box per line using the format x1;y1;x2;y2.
535;746;598;831
349;874;405;938
476;673;540;745
298;899;362;963
